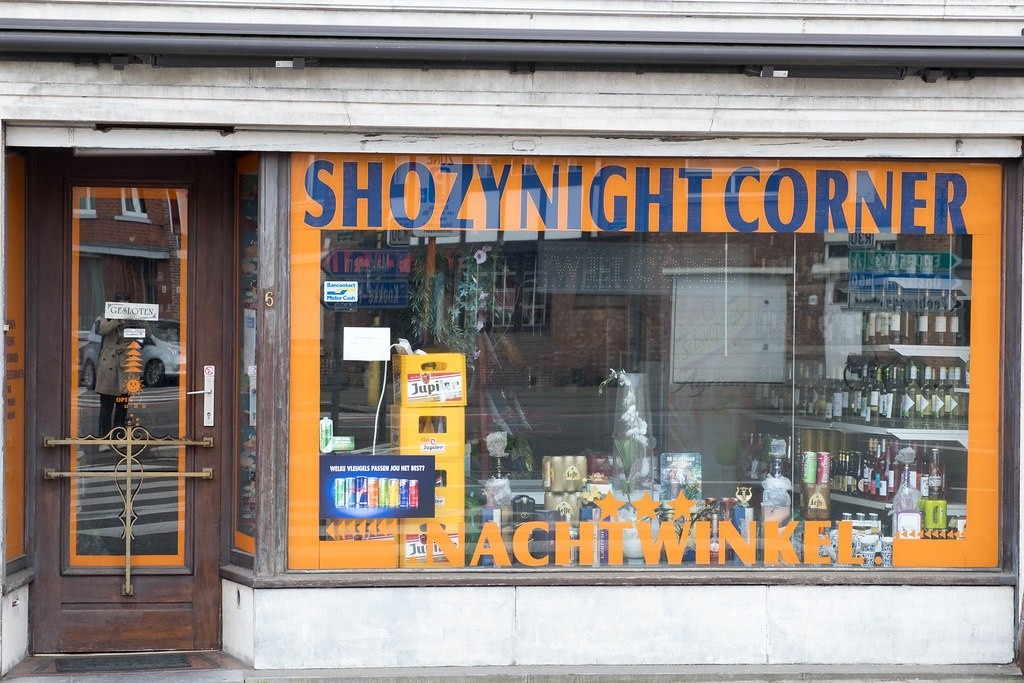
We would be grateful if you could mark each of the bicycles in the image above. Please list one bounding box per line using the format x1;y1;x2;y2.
843;332;912;394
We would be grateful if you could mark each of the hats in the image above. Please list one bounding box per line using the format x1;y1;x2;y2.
114;291;131;300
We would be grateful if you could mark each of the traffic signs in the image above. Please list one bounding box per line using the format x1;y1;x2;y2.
320;277;410;312
320;246;412;279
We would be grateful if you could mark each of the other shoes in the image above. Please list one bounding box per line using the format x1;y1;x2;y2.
116;444;127;449
99;444;111;454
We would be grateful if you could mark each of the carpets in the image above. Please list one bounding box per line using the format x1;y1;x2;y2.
56;653;190;674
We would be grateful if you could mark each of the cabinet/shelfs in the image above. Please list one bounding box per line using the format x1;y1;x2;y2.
753;277;971;514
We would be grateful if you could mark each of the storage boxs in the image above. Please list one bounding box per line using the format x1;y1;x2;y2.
319;352;466;565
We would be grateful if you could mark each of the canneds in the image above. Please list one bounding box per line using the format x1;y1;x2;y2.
542;455;588;524
704;496;731;538
800;451;830;485
772;458;781;477
334;476;419;508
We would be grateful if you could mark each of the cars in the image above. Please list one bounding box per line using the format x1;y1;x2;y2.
72;330;92;386
83;314;180;391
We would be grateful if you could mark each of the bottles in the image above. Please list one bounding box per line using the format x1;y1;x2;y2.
737;278;969;531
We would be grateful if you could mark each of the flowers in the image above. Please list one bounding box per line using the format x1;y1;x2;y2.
411;237;499;370
598;369;651;511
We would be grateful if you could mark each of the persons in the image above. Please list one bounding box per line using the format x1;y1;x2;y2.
95;291;152;453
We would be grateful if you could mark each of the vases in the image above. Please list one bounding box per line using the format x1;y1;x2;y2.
606;373;658;522
